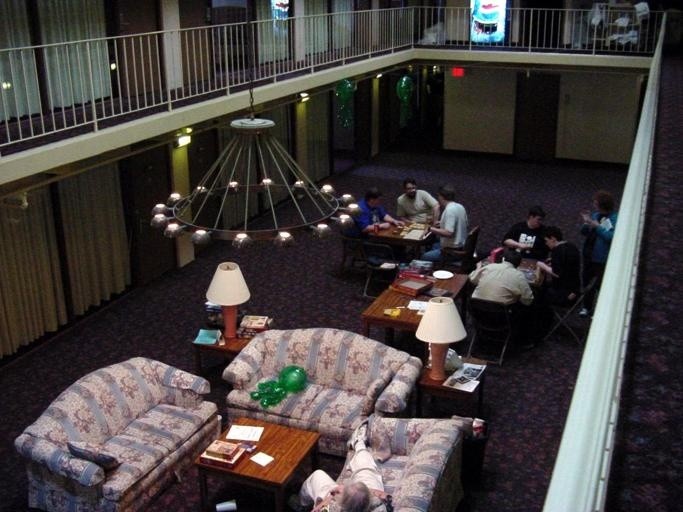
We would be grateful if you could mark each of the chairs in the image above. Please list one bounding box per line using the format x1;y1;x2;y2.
536;249;601;347
464;218;565;331
467;296;522;365
336;202;479;296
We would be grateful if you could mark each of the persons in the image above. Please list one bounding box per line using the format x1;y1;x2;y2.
420;183;468;263
467;249;535;352
535;225;580;305
501;205;550;262
299;418;395;511
345;187;403;261
575;189;617;317
397;177;441;227
457;376;464;383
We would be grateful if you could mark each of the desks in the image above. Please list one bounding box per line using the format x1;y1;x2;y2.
413;357;487;418
190;414;322;510
360;269;471;359
190;330;258;408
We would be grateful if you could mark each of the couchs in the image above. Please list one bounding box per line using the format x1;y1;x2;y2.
12;356;223;512
307;412;467;511
219;327;421;463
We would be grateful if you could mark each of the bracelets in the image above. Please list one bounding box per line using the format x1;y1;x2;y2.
427;226;430;232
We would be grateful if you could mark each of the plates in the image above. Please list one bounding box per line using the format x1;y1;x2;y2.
432;270;453;280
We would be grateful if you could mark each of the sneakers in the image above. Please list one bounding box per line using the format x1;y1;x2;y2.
346;420;367;450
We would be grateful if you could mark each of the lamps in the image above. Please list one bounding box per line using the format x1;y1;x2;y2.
413;296;470;380
146;0;364;253
201;261;251;339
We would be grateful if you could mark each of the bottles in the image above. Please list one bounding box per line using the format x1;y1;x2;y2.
273;1;288;29
472;0;501;34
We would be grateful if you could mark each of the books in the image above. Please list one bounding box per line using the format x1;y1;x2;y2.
194;297;272;345
199;440;245;470
205;440;239;460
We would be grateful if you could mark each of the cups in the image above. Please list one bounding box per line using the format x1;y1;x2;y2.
215;499;237;511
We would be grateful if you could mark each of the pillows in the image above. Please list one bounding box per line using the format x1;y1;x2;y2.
63;437;125;473
